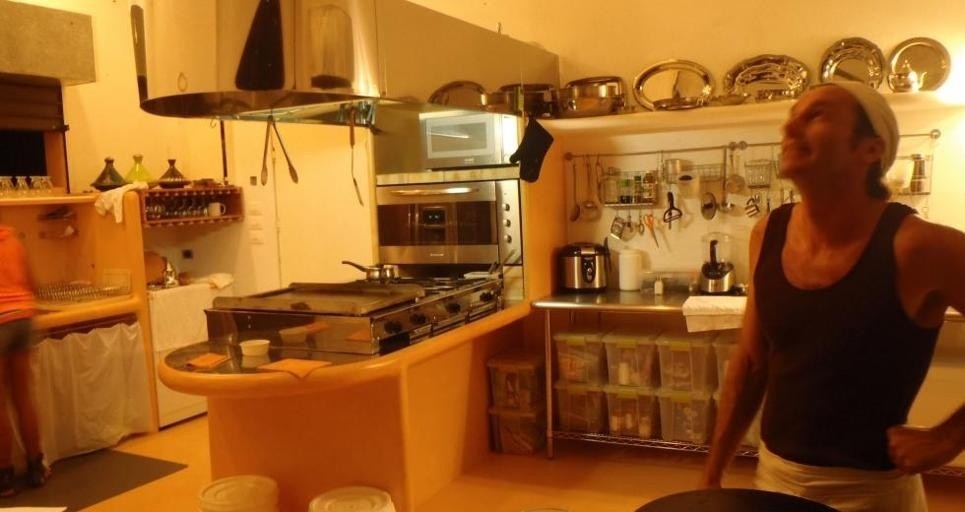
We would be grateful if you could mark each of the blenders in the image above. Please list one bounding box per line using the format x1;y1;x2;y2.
701;232;735;294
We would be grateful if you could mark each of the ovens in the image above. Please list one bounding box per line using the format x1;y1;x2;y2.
373;178;523;266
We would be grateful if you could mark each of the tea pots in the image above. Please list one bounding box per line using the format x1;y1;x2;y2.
887;60;927;92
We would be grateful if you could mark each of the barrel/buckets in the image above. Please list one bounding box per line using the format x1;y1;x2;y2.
307;485;395;512
197;474;279;512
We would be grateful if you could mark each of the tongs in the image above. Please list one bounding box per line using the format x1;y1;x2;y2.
261;115;299;186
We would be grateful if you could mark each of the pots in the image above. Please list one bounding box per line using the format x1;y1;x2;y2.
342;260;401;282
490;83;560;119
561;76;628;116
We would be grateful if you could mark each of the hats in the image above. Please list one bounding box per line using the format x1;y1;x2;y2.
811;80;900;176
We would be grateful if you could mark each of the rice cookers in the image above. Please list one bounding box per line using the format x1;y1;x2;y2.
557;242;610;294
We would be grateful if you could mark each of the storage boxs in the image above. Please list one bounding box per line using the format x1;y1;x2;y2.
553;323;760;444
491;341;545;460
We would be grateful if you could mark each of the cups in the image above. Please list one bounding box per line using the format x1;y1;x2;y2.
665;158;681;175
887;159;915;193
146;198;226;220
744;159;772;189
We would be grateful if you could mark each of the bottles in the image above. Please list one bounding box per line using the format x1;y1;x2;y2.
0;176;56;199
604;166;657;203
163;249;195;288
618;248;664;295
89;153;191;192
909;154;925;192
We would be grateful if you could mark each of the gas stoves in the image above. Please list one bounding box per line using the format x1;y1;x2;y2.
204;266;504;356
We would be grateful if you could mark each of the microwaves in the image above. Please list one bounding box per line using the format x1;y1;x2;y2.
419;111;520;168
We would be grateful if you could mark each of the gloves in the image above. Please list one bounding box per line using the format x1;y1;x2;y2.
510;117;553;182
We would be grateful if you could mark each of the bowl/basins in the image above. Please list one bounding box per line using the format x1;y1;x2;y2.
886;37;951;91
632;54;810;112
427;80;490;109
239;339;270;356
278;326;307;345
817;37;887;92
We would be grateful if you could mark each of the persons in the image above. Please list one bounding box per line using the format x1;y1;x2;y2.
698;79;964;511
0;222;57;499
